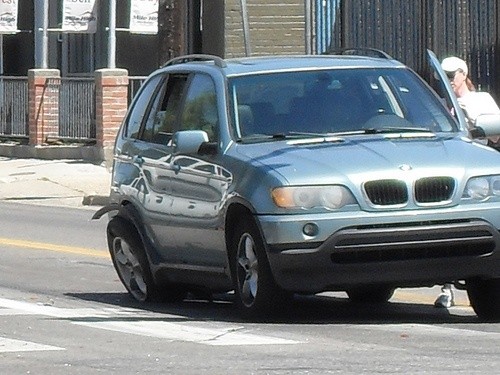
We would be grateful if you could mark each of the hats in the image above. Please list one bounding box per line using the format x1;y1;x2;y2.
433;56;468;80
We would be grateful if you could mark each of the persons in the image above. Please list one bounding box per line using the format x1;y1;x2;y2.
431;57;500;308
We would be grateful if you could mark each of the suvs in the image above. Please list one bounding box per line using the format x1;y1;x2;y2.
90;46;500;321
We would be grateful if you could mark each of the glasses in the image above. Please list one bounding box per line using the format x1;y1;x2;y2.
446;69;458;79
